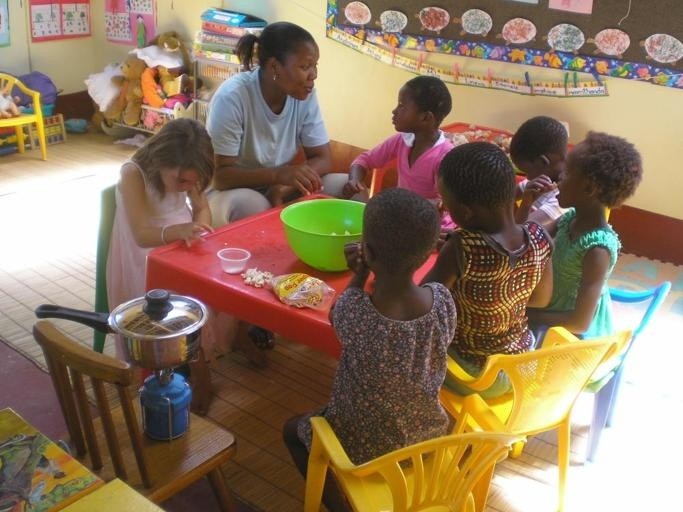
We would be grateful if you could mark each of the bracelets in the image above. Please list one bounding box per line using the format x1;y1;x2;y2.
199;231;209;236
160;224;171;245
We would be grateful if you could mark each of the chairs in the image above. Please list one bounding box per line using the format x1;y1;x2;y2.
32;319;236;512
91;183;117;352
585;281;671;462
0;73;47;160
303;393;515;512
440;327;630;510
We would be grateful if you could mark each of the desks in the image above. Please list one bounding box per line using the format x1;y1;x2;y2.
144;195;465;361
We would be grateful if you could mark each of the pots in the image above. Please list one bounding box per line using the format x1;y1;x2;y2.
36;289;211;366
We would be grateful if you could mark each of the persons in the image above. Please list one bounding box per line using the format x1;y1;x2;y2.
106;118;271;416
189;22;364;233
514;130;643;350
342;76;461;232
509;116;574;243
0;90;20;119
134;15;145;48
416;142;555;399
281;188;457;511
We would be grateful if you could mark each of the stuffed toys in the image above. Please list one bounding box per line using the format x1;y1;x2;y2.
91;30;202;134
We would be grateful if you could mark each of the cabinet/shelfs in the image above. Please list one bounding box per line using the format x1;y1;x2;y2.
99;101;195;139
192;56;256;123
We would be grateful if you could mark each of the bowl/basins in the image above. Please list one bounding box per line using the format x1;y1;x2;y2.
218;248;251;274
279;198;367;268
